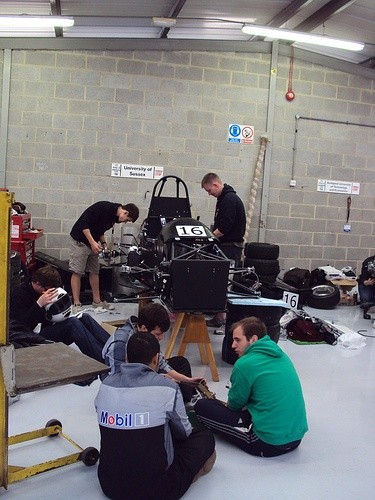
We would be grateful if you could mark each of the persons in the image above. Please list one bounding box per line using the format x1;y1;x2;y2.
201;172;247;327
356;255;375;308
9;266;111;364
68;201;140;309
100;302;207;404
192;316;310;457
93;330;217;500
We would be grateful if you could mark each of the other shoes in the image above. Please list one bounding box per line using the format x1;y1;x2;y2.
92;301;116;310
205;317;226;327
213;324;225;335
73;303;85;315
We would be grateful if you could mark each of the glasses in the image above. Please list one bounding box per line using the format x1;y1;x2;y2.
42;285;48;291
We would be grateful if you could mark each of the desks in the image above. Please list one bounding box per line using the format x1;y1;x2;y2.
130;272;229;382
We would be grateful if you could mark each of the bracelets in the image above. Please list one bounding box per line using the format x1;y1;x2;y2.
101;242;107;244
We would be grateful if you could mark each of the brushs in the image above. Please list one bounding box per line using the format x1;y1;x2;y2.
343;195;352;232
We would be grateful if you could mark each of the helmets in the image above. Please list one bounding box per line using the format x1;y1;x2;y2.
43;287;72;325
